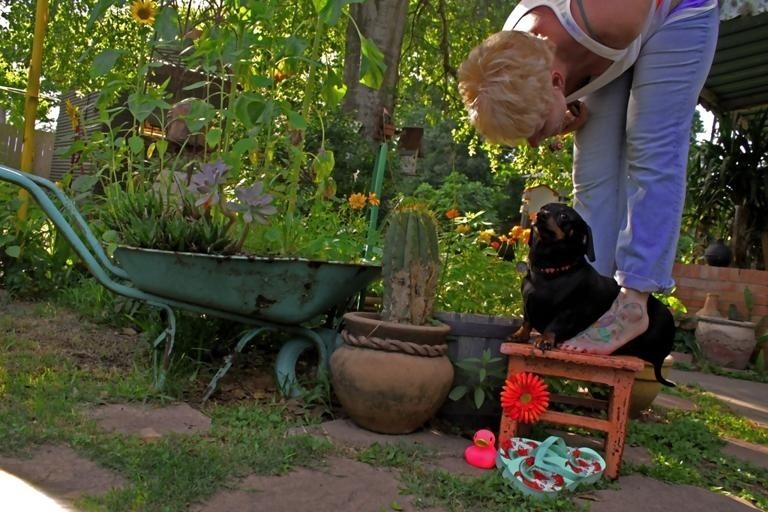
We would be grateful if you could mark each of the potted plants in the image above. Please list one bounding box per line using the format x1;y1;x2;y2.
329;203;454;436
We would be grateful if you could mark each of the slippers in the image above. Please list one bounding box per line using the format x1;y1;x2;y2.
497;434;606;498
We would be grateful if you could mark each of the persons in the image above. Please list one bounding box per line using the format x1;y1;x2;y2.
457;0;720;357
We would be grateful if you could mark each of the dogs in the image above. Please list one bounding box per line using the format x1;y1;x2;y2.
507;201;677;389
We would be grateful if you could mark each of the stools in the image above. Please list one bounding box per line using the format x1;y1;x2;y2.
498;342;644;482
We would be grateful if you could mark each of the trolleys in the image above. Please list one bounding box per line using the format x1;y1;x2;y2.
0;166;383;401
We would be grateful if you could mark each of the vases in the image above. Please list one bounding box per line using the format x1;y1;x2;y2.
629;354;673;419
694;314;757;370
705;238;730;266
435;310;521;417
696;293;720;318
